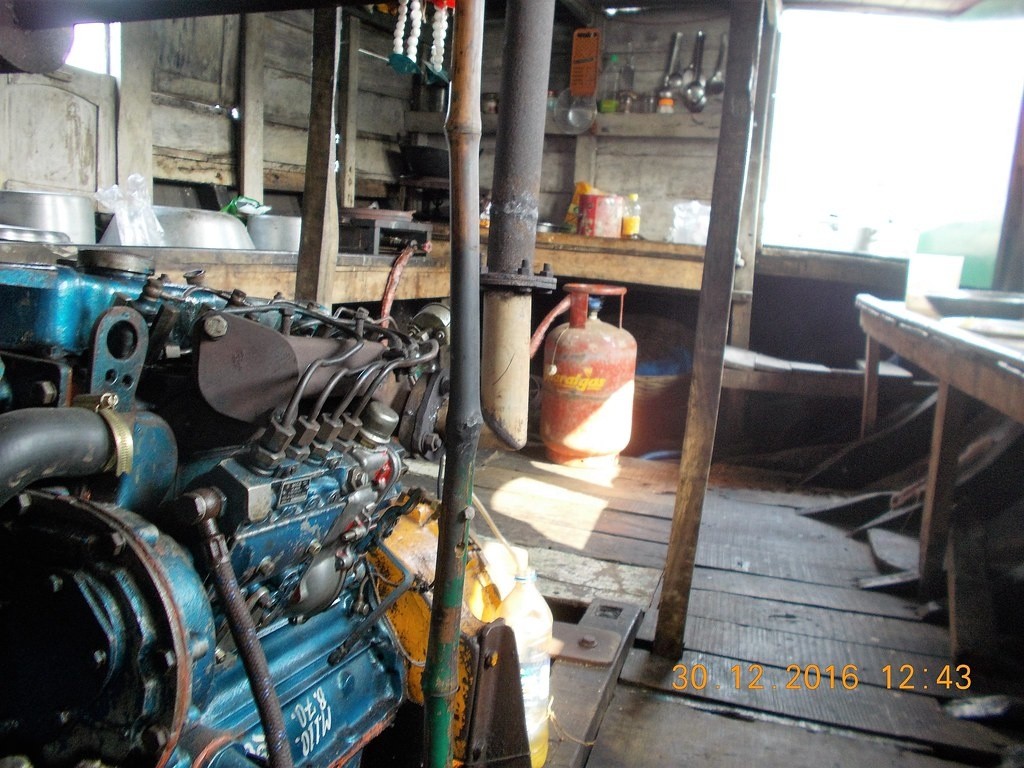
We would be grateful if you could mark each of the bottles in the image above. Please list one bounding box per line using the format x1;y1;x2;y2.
600;54;621;112
483;93;497;115
622;194;641;241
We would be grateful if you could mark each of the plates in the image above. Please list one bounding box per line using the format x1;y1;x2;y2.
940;315;1024;337
923;288;1024;319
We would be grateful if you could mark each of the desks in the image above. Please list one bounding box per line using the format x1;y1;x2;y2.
754;244;910;370
430;223;744;290
0;241;451;304
856;293;1024;600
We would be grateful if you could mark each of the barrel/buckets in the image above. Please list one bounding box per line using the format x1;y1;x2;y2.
0;191;97;244
246;214;302;252
